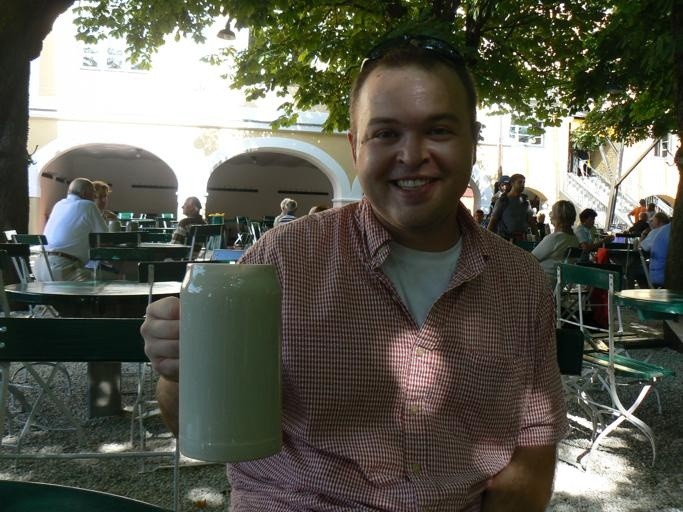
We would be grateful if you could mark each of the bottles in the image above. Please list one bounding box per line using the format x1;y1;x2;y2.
525;227;532;241
176;263;283;464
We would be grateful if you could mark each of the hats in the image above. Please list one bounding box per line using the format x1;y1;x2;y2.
499;175;511;185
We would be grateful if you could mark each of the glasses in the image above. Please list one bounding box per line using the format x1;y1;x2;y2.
359;36;464;74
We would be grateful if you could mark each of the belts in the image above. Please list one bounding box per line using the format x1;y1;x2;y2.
45;251;76;261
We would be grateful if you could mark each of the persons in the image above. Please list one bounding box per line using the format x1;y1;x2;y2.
578;146;588;176
139;38;573;512
629;199;671;287
273;198;298;227
474;174;550;252
34;178;117;282
533;200;614;274
172;196;208;243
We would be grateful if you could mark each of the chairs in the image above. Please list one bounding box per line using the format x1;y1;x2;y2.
554;229;675;471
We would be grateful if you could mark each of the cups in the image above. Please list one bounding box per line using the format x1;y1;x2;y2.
596;247;607;265
106;219;140;247
